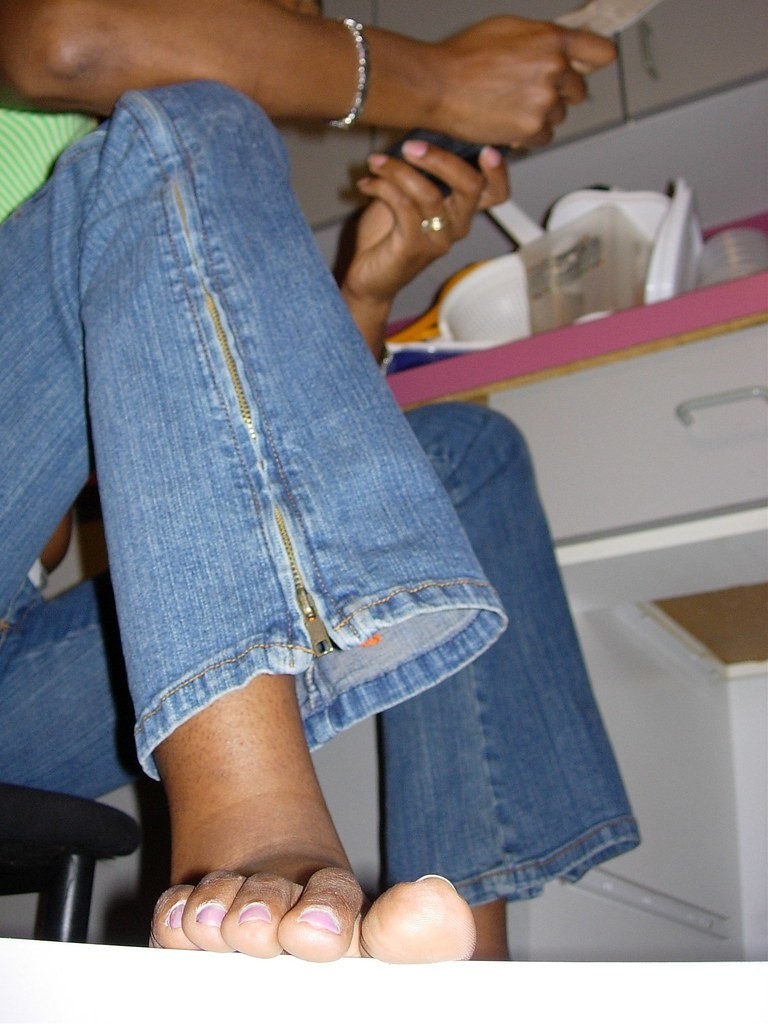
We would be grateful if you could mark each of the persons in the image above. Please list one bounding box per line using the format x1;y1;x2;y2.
0;0;641;963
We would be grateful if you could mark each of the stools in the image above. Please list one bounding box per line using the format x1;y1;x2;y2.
0;783;146;945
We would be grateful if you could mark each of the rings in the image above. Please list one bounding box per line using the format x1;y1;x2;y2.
421;217;447;232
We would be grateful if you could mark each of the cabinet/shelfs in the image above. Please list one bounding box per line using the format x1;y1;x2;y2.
277;0;768;231
108;268;768;961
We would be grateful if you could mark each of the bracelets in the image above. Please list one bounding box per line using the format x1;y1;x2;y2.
328;17;370;129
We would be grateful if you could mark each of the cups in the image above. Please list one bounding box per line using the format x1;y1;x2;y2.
518;204;653;333
